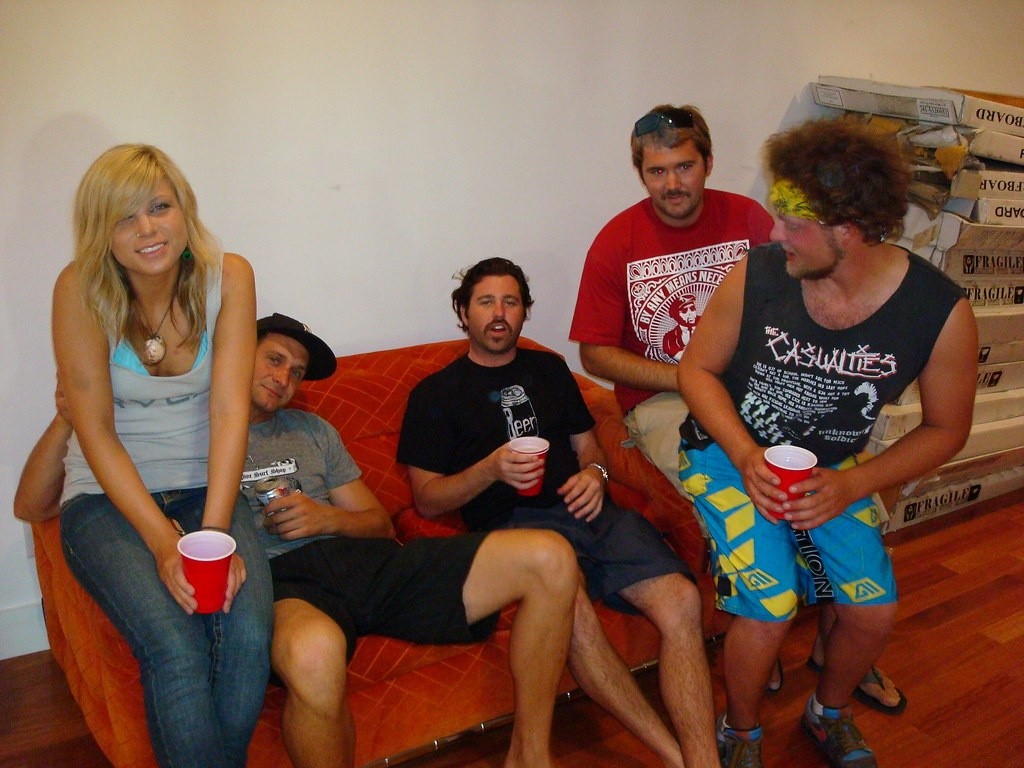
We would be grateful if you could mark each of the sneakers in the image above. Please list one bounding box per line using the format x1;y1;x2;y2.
800;696;877;768
715;710;764;767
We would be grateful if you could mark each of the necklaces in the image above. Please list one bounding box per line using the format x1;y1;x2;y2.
138;296;174;366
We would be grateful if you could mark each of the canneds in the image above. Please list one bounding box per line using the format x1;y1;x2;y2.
256;476;291;526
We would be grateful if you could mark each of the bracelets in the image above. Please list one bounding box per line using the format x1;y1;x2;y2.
585;462;608;485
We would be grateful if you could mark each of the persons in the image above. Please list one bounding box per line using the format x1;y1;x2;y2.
395;257;721;768
567;103;776;506
12;314;579;768
52;144;272;768
674;117;980;768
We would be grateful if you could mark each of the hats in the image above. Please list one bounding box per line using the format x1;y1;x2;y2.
255;314;337;381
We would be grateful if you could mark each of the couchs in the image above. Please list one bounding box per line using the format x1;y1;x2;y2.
30;336;737;768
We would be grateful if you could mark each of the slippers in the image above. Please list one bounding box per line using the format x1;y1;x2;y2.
763;658;784;693
805;655;907;715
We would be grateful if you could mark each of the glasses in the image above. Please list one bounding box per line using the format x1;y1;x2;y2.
634;110;695;137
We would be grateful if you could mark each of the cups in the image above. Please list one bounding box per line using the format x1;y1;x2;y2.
763;445;817;518
177;529;236;613
508;438;549;496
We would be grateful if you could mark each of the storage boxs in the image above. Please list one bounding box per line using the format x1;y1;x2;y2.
809;73;1024;540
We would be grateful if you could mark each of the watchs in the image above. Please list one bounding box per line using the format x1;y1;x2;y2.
200;526;232;535
